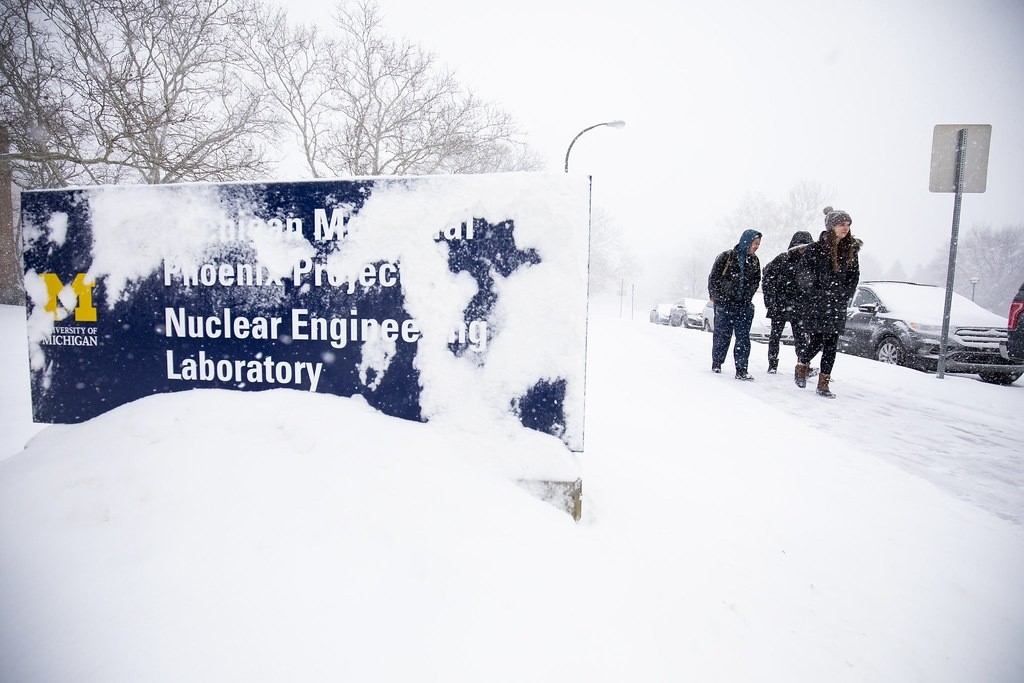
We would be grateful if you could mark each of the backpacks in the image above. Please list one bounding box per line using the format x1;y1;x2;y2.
794;243;824;295
708;249;734;303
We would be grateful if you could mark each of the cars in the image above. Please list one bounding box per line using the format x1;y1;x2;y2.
703;300;715;333
670;298;706;330
745;292;797;344
650;302;674;324
840;280;1024;386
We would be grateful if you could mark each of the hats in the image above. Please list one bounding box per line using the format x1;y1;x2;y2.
822;205;853;229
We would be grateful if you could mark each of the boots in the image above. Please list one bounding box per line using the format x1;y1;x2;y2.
815;373;836;399
794;363;808;388
766;356;779;374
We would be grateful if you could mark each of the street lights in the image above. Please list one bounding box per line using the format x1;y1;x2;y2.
564;119;625;174
969;278;981;303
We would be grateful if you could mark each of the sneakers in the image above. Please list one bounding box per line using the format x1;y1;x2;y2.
712;361;722;373
734;367;755;381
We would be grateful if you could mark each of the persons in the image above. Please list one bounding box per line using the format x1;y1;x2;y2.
794;206;864;398
707;228;763;381
762;230;819;377
